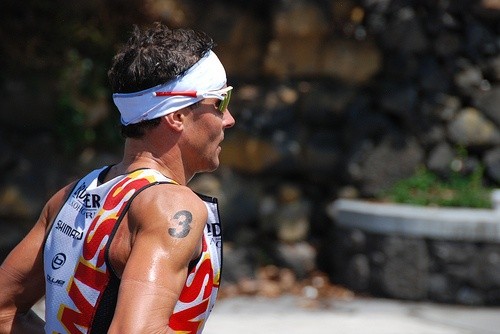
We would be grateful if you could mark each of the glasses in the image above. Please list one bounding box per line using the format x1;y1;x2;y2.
152;85;234;113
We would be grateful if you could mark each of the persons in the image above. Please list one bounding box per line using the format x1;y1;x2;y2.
0;24;237;334
